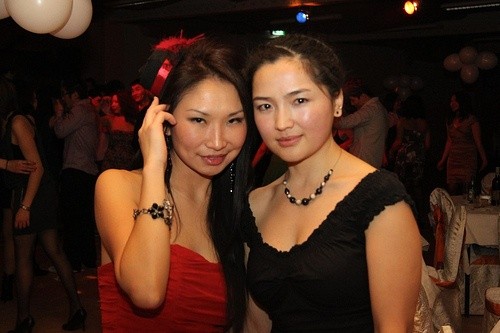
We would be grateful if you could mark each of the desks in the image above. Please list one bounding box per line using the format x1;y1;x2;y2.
450;196;500;320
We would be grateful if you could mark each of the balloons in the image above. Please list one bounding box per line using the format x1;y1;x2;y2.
397;87;412;102
383;76;399;89
3;0;73;35
444;47;498;83
49;0;92;43
410;79;423;91
0;0;10;19
399;74;411;87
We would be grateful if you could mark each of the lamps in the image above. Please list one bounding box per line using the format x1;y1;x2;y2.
295;9;311;24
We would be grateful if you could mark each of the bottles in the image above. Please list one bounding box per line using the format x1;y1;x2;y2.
491;167;500;206
467;176;475;202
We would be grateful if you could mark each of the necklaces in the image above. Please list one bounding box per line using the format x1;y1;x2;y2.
283;168;333;206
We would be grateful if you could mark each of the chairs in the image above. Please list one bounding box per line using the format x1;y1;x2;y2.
412;173;500;333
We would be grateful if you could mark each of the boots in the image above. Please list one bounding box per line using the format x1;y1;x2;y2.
33;264;49;276
0;271;14;303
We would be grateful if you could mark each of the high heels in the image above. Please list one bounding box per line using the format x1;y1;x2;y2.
7;313;35;333
62;307;88;332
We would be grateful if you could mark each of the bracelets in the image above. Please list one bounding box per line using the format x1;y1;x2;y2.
133;199;174;230
19;203;32;211
441;159;445;162
6;160;9;171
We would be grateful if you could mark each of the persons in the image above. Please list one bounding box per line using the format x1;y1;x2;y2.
94;40;253;333
0;66;487;333
242;35;422;333
